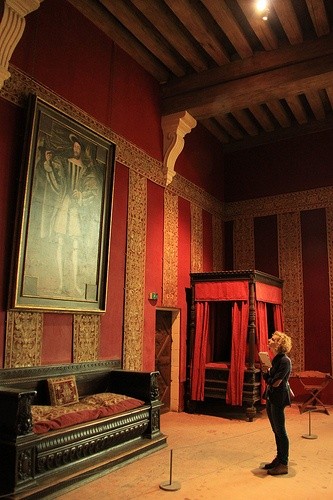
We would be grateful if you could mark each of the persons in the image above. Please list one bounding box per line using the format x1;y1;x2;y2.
258;331;293;477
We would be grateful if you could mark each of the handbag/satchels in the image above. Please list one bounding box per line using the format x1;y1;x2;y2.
289;388;295;401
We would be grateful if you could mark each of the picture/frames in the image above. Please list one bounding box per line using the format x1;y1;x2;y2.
4;95;118;317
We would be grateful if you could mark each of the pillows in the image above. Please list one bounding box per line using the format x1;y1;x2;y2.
46;374;80;407
0;368;168;500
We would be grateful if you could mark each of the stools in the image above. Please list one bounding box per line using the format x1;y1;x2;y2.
295;371;332;416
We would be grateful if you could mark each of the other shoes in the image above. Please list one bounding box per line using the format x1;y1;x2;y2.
265;470;281;470
267;463;288;475
